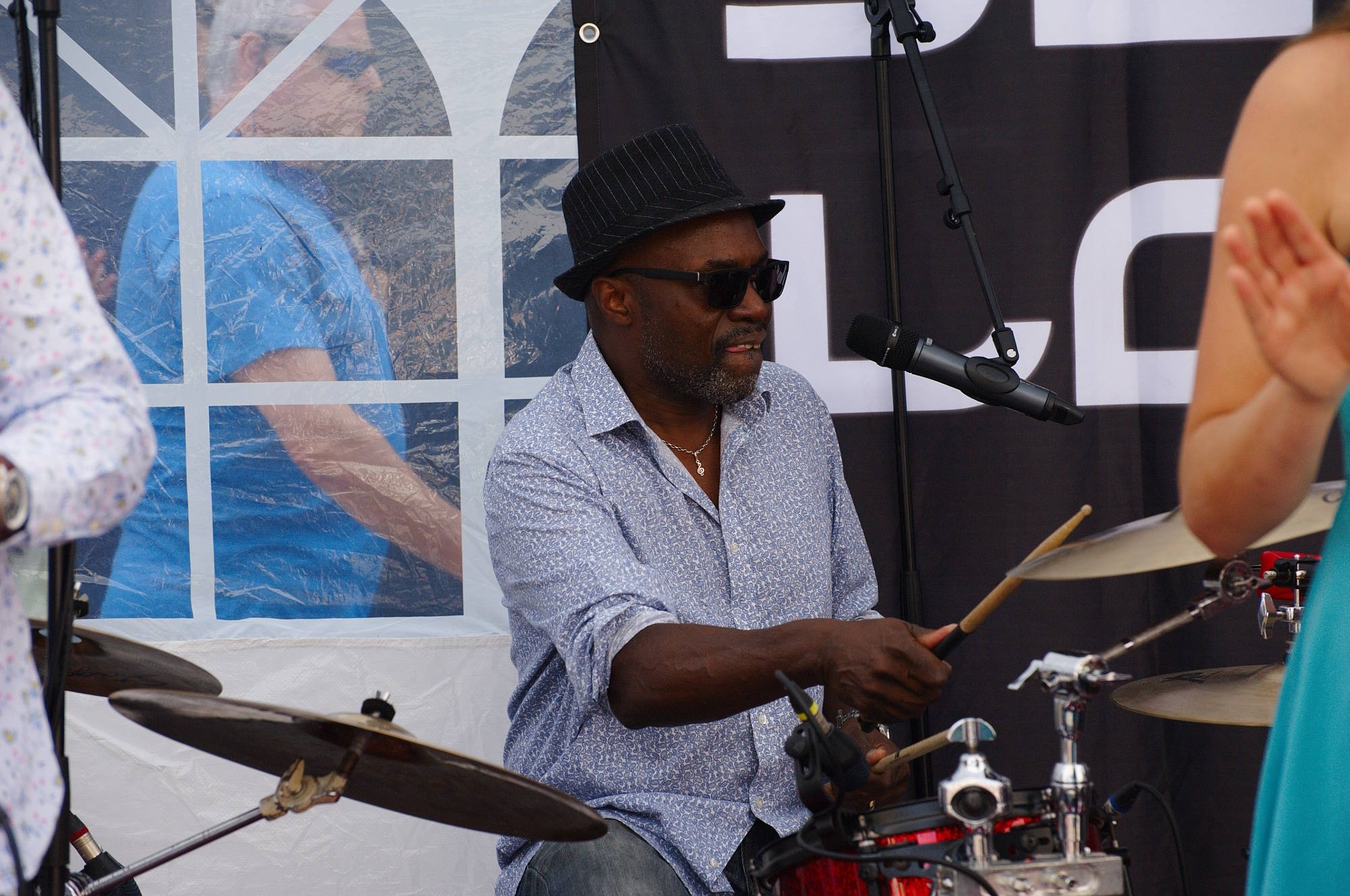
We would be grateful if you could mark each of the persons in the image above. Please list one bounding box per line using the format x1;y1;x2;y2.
1181;30;1350;896
484;123;952;896
100;0;461;617
0;80;159;896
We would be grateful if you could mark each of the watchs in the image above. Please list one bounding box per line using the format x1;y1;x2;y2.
0;464;28;531
836;709;890;740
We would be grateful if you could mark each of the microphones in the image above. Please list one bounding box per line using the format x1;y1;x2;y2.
784;682;870;792
70;811;142;896
845;312;1085;425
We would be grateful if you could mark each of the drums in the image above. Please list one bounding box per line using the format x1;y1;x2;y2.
749;787;1107;896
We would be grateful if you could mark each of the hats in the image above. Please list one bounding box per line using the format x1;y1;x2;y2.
553;124;785;301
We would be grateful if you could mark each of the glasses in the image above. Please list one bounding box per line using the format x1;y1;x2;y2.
232;28;388;81
582;258;790;309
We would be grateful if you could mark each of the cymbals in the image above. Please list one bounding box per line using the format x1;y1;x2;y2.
1111;661;1288;728
110;687;610;845
1004;478;1348;584
26;614;224;701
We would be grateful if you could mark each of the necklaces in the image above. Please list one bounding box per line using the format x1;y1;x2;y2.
662;405;718;476
